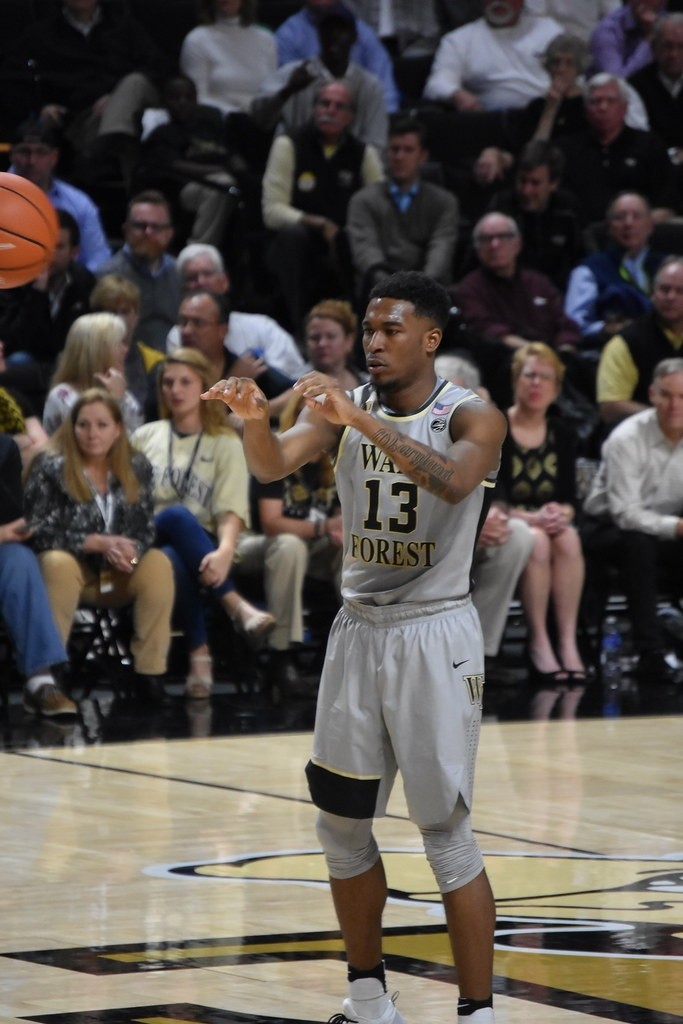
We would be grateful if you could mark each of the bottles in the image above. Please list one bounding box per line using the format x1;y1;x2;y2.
600;613;624;672
601;673;621;717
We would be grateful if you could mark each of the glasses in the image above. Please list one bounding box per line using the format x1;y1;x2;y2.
479;232;514;243
127;222;174;233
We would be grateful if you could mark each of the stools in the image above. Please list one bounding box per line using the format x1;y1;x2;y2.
74;602;141;698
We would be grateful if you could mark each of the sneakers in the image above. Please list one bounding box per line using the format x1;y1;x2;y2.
327;992;407;1024
23;684;78;716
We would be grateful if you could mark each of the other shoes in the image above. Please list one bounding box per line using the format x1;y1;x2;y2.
232;601;276;651
186;655;214;697
140;677;179;710
637;648;683;683
268;653;311;694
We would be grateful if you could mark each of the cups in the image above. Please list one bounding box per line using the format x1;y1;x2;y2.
111;539;143;587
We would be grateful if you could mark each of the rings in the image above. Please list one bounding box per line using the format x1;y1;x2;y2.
130;556;139;565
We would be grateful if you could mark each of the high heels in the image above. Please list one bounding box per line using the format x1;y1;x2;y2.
554;649;587;682
524;647;570;683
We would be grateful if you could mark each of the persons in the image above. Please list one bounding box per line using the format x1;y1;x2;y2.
1;0;683;721
200;271;509;1024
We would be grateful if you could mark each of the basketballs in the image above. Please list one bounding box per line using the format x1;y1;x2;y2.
0;172;60;289
